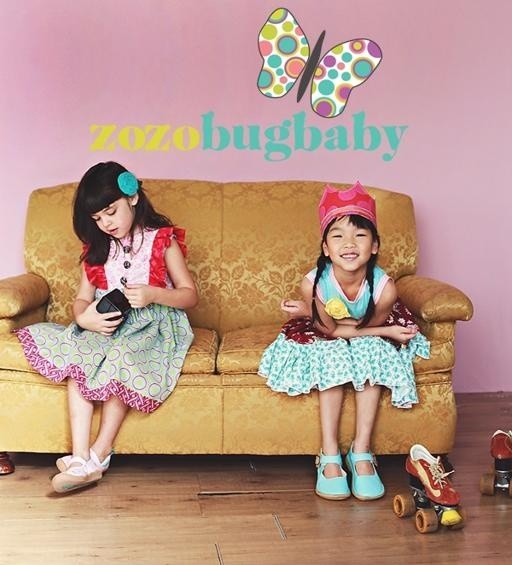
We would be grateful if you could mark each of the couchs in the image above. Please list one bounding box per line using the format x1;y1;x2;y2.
0;178;474;476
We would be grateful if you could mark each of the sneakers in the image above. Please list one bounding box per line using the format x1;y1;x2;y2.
53;448;111;493
346;444;386;500
313;448;350;501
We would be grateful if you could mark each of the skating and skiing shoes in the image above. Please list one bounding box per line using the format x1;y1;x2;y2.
479;428;511;495
395;444;466;535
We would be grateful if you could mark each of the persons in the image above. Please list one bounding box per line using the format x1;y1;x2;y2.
10;160;199;494
256;179;431;502
